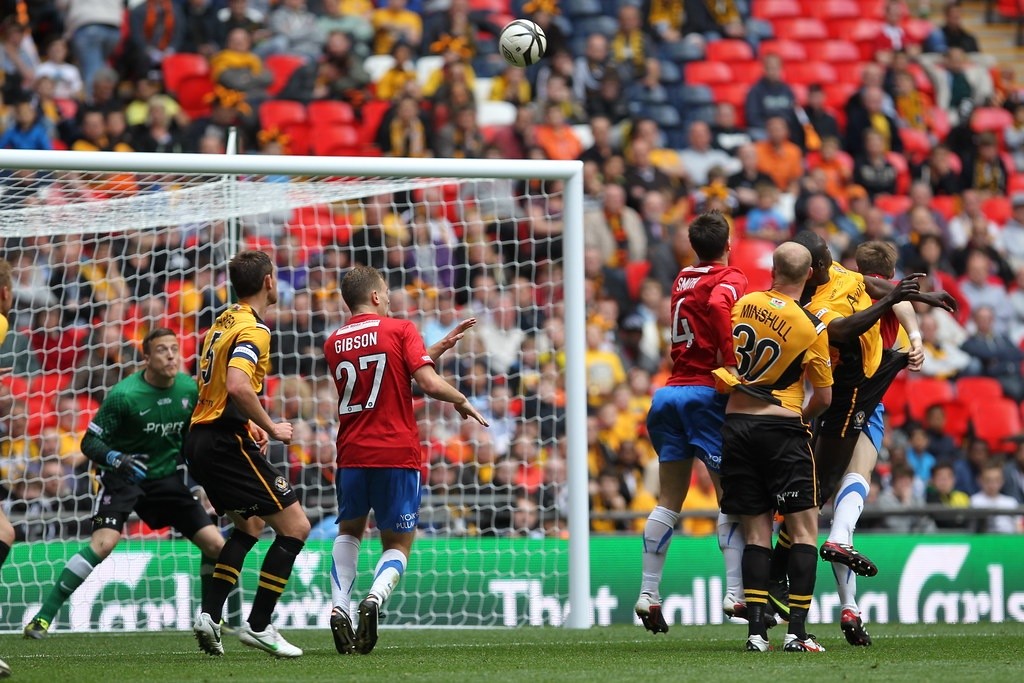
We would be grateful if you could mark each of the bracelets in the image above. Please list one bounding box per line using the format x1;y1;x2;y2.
907;331;921;342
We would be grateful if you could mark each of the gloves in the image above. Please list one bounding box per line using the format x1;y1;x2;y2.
107;450;149;485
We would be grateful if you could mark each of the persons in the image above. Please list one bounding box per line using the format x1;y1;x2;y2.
183;249;311;661
22;326;269;641
322;268;489;654
0;257;16;679
0;0;1024;537
635;208;955;651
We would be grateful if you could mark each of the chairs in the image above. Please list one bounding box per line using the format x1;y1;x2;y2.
883;368;1024;454
163;50;384;156
682;0;911;132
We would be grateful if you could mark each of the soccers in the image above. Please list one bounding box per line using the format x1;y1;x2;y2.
496;18;547;68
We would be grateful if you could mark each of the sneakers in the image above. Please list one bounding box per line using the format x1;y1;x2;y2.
819;541;878;578
783;633;826;652
635;592;668;635
239;620;303;660
330;606;355;654
767;577;791;621
193;612;224;656
351;593;381;655
723;593;778;629
745;634;775;652
841;609;872;646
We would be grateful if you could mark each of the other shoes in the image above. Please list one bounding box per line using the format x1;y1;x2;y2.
24;618;47;640
0;659;13;677
